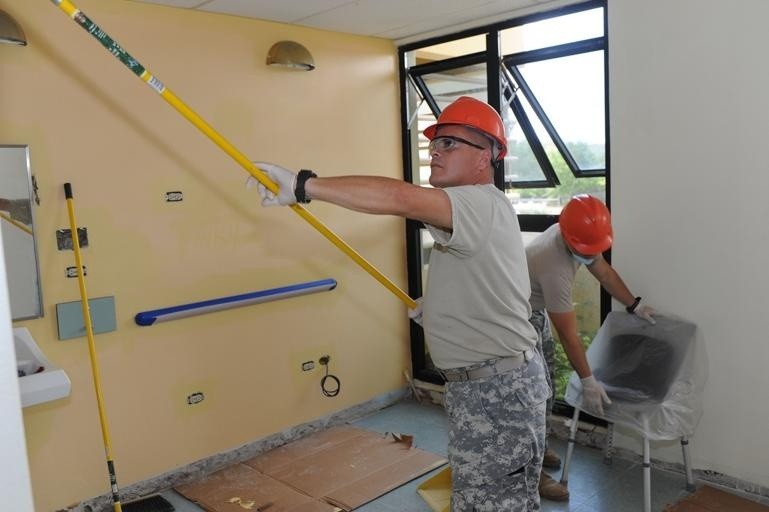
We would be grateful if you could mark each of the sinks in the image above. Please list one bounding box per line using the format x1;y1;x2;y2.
16;362;70;407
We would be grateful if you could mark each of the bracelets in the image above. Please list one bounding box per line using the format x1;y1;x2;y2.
626;296;642;314
294;168;318;204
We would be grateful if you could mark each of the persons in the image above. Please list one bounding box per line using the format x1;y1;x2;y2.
246;96;551;512
524;193;662;500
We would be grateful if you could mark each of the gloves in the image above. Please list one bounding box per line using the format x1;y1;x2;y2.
579;373;612;419
632;300;666;324
244;162;297;208
407;294;426;329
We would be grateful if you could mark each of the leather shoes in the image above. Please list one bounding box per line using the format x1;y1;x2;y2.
534;469;572;502
543;446;561;467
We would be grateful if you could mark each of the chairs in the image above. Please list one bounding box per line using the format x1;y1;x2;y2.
552;308;706;512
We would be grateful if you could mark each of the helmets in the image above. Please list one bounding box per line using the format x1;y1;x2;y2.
422;94;507;162
558;191;615;256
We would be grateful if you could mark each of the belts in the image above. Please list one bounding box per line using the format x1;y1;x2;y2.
438;342;540;391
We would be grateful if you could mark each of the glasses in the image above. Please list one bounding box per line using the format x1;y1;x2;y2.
426;135;487;156
